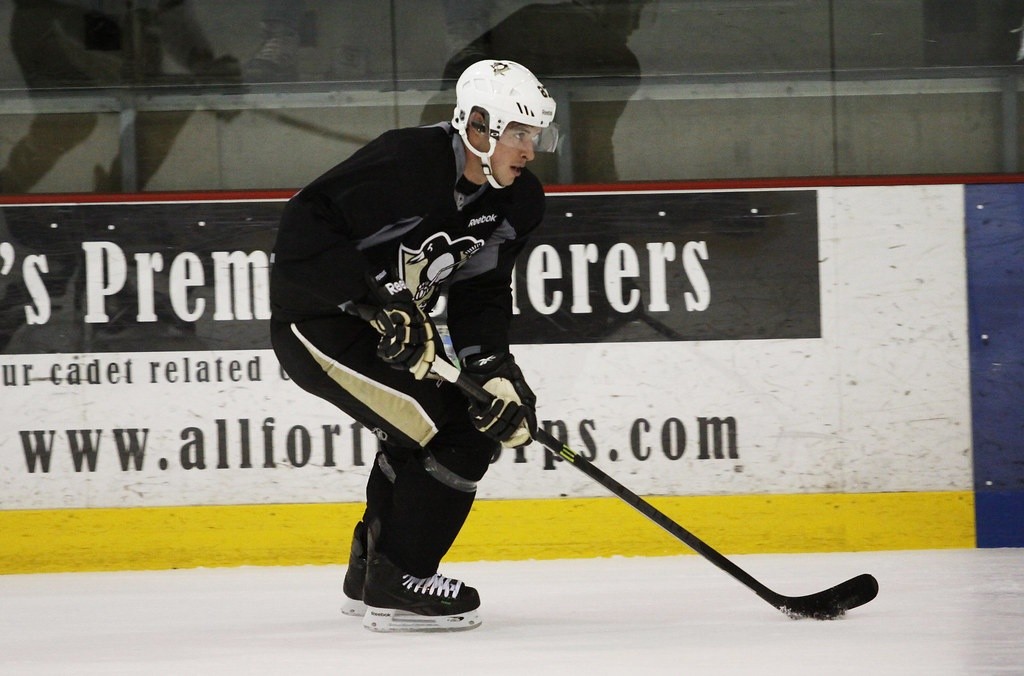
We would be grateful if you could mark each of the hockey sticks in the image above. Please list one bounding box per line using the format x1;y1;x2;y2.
430;354;880;624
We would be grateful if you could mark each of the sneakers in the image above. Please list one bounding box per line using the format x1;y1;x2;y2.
343;521;480;615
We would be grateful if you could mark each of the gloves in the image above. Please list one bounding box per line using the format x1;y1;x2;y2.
354;271;435;381
459;346;539;449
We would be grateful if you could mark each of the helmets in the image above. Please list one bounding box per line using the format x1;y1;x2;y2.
452;60;562;158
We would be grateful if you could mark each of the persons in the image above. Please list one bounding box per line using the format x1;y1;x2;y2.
270;59;560;633
